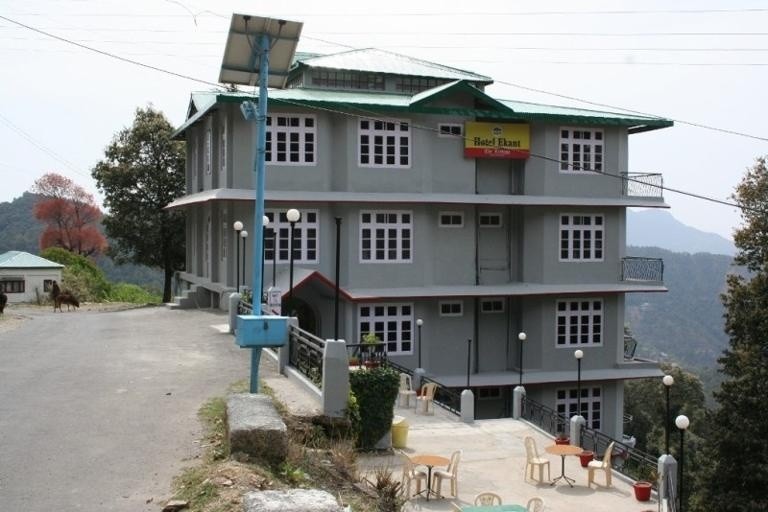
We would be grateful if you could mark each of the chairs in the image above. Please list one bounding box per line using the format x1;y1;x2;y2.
398;372;437;416
399;436;616;512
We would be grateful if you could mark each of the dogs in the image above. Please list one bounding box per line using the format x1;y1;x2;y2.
54;294;79;312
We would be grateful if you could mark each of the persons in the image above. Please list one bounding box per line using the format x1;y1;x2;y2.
52;280;60;308
0;292;7;311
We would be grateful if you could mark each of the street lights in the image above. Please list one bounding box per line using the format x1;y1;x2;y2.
573;349;585;419
232;212;269;311
416;319;426;377
517;332;528;387
285;208;302;318
662;374;674;457
673;415;690;512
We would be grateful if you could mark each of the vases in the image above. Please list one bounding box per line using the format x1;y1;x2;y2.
634;481;652;501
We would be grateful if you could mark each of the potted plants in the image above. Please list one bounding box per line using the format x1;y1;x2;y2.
361;333;380;369
